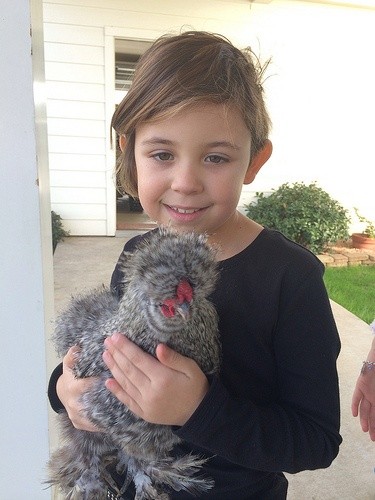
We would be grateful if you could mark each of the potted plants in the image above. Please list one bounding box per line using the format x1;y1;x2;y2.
352;207;375;250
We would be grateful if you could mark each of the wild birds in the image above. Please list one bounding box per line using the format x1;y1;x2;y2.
44;223;220;500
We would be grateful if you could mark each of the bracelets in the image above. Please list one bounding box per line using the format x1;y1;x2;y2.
360;360;374;376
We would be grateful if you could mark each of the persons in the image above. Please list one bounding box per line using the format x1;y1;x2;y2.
351;318;375;441
46;31;344;500
